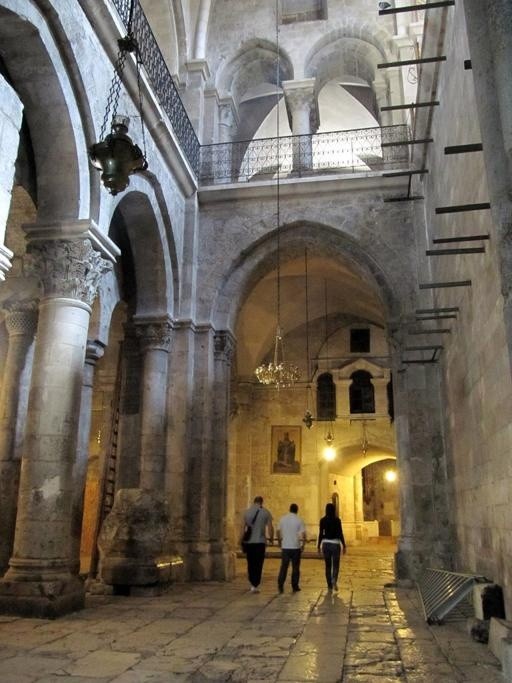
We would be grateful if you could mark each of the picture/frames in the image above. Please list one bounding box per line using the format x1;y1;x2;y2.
270;423;302;476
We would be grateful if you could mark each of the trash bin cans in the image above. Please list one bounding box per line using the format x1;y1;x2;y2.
472;583;505;643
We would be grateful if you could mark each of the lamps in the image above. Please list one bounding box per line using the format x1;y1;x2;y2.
255;0;300;388
302;244;373;456
88;0;149;197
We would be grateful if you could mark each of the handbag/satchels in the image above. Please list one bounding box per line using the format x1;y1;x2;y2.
245;525;252;541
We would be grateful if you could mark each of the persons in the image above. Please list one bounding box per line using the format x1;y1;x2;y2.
239;496;274;593
318;503;346;593
276;503;307;593
277;432;295;464
